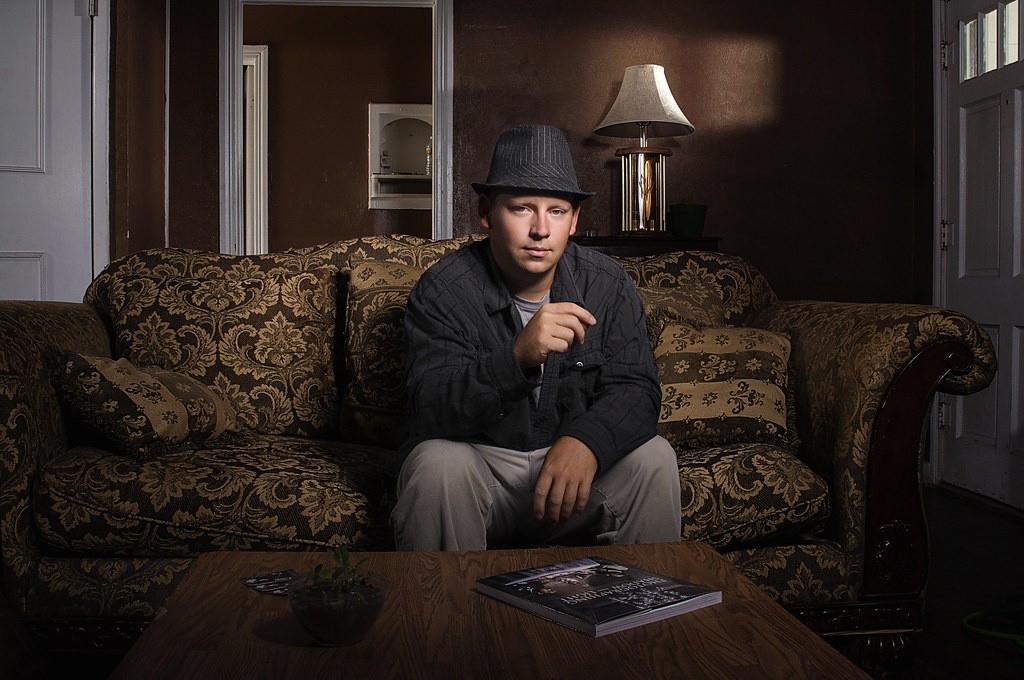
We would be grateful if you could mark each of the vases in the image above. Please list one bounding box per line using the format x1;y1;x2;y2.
670;204;707;240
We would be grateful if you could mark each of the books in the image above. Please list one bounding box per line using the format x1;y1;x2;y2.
475;556;723;640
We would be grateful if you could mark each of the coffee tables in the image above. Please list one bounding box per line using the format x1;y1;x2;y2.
109;540;870;680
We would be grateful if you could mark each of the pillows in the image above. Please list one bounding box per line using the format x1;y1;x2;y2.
341;258;428;449
109;267;343;439
653;320;792;446
635;281;726;349
48;344;259;461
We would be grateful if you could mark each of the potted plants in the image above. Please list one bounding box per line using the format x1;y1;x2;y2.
288;546;392;648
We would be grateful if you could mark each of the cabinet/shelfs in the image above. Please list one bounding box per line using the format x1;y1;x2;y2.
571;235;723;257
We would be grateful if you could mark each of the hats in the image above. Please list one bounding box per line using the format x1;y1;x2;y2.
470;124;595;202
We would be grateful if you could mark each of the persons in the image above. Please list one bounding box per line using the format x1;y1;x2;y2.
390;124;681;555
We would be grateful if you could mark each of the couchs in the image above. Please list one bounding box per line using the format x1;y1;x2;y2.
0;233;1000;680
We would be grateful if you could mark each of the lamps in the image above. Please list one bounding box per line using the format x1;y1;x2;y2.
592;65;695;240
